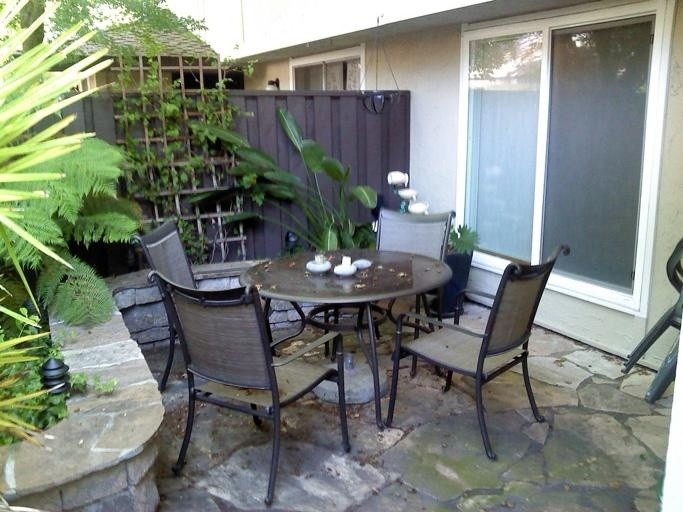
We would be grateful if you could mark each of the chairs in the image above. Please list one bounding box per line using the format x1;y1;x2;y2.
330;208;457;379
620;237;683;407
146;269;351;506
381;244;563;462
137;217;276;394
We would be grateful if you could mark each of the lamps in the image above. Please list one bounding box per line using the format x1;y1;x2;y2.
265;78;278;92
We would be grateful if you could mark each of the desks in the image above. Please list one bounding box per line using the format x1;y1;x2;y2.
239;249;454;404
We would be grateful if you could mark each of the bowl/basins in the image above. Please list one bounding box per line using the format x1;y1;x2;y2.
306;259;332;274
333;264;358;277
